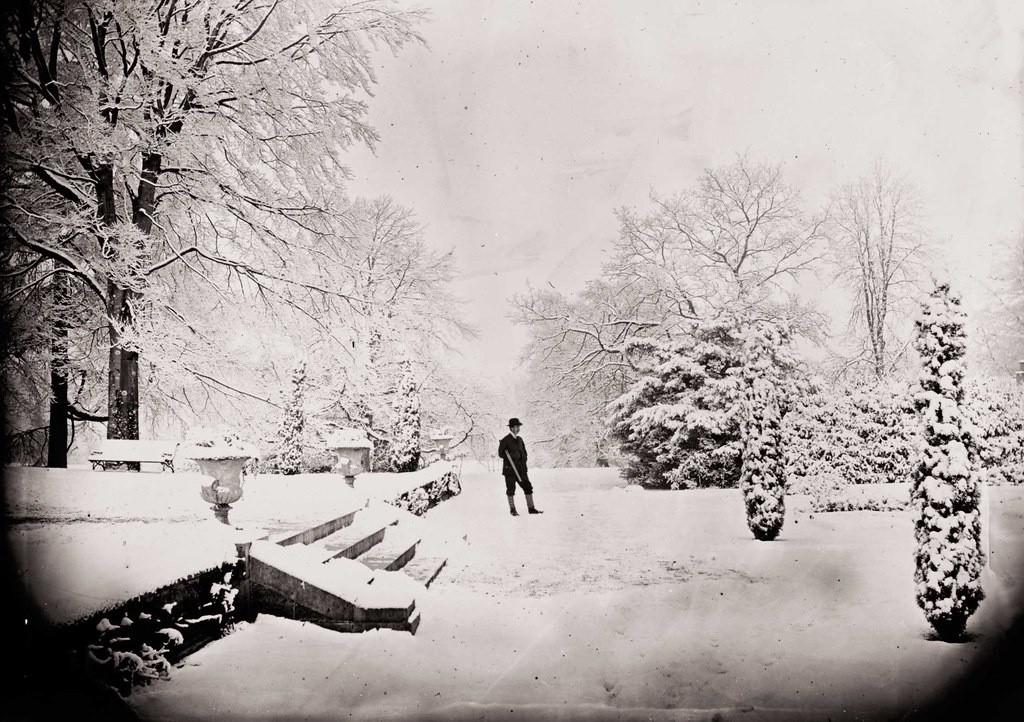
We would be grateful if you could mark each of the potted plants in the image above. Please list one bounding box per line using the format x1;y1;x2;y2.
431;434;454;460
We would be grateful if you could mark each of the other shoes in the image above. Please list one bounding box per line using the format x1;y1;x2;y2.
529;507;543;514
510;508;519;516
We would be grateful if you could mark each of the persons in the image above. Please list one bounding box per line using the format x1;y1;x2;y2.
498;418;543;516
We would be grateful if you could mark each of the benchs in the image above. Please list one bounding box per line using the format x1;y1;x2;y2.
88;440;181;474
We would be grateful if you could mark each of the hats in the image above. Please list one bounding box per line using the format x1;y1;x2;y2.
507;418;523;426
